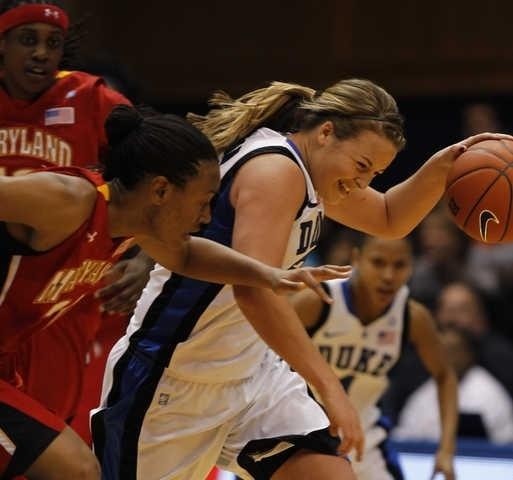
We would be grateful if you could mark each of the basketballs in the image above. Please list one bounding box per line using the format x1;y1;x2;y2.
445;138;513;248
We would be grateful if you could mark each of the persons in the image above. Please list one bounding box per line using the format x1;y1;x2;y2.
299;94;512;446
85;76;513;480
289;227;461;479
0;377;104;480
0;0;155;450
0;99;355;480
